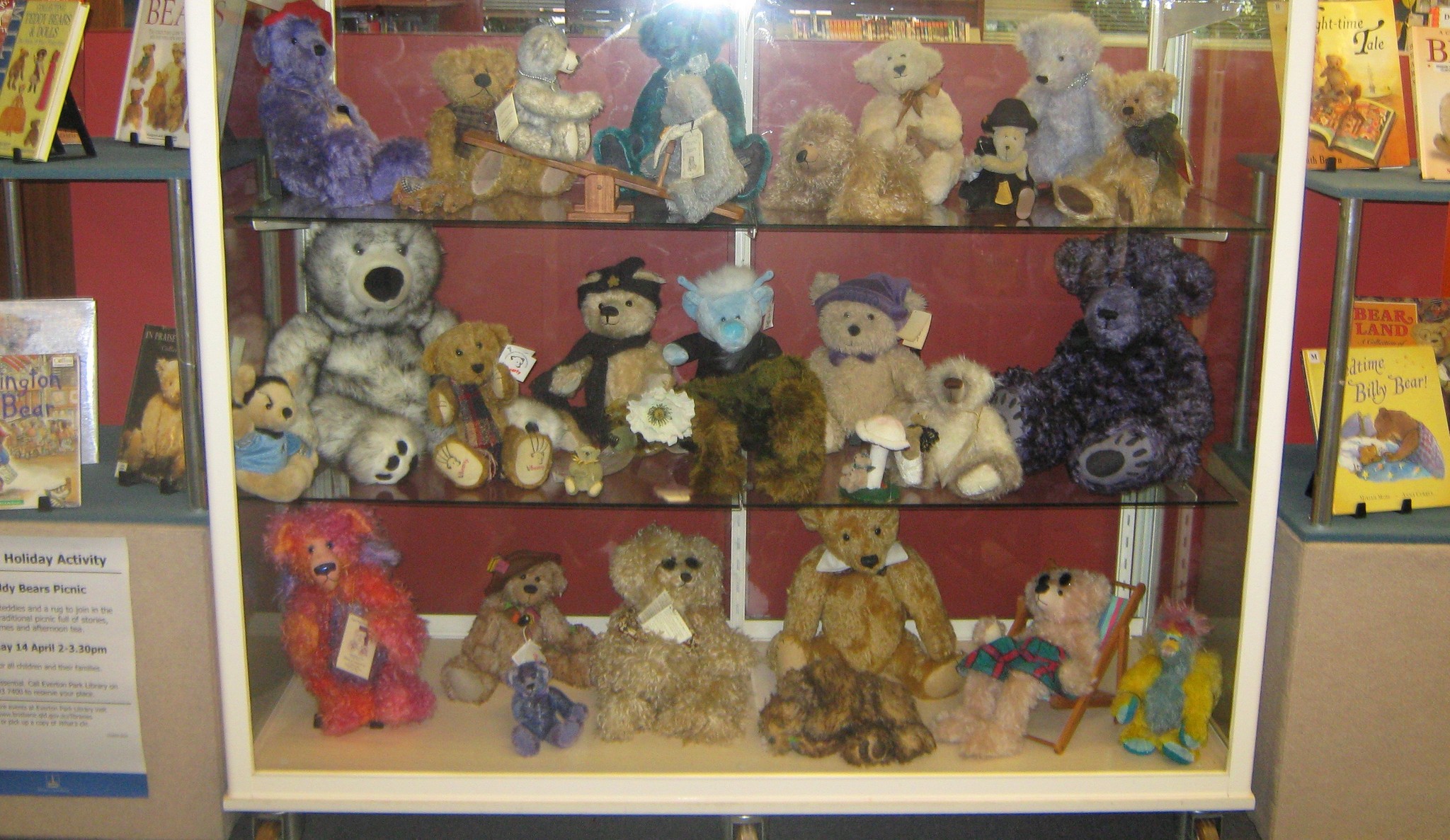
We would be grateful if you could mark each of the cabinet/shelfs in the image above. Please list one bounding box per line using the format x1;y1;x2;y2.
1214;149;1445;543
183;0;1319;839
0;136;278;524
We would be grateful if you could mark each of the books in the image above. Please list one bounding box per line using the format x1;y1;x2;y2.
1267;0;1450;182
335;15;980;43
0;0;90;163
1299;292;1450;515
0;298;245;510
114;0;246;148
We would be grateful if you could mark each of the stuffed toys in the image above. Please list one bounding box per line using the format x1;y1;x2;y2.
262;504;435;737
766;507;967;699
760;103;925;224
590;521;759;746
928;568;1110;762
1053;62;1195;226
231;8;1219;763
807;272;927;453
989;234;1216;495
